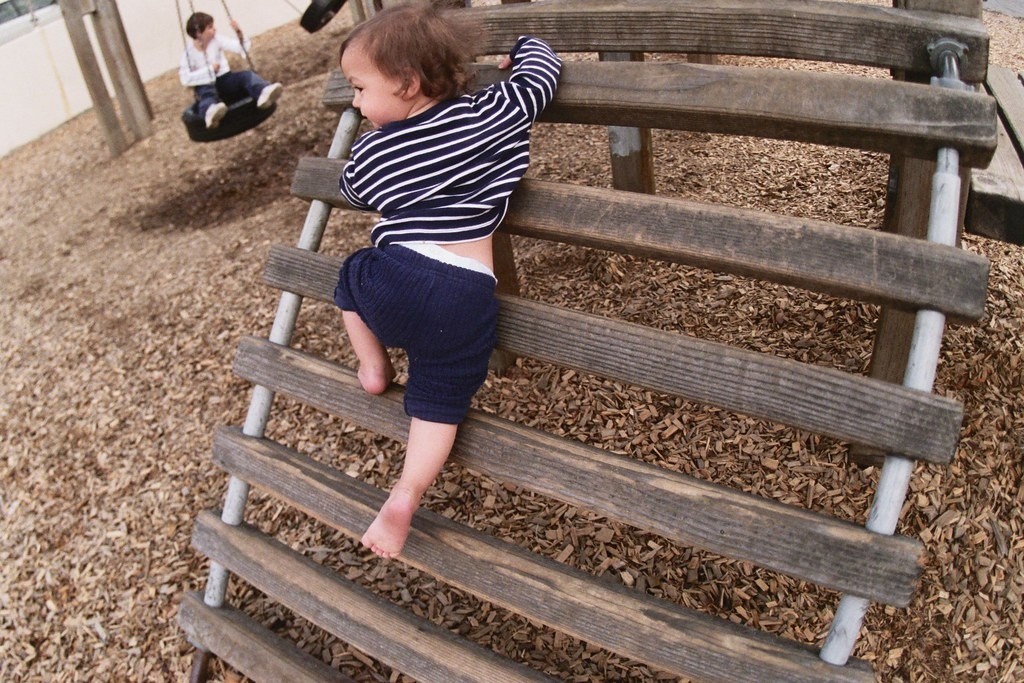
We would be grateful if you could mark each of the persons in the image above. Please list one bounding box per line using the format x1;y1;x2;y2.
339;4;563;559
179;11;284;128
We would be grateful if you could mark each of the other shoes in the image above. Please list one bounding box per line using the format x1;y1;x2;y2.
205;102;227;129
257;82;284;109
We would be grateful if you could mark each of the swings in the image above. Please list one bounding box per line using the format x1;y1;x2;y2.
283;0;347;33
175;0;279;142
